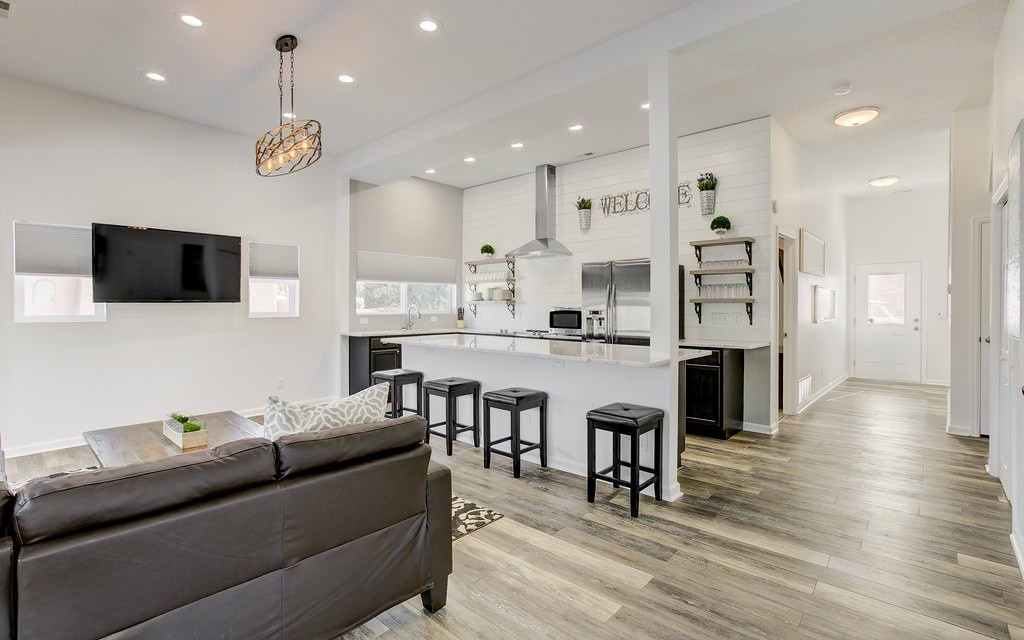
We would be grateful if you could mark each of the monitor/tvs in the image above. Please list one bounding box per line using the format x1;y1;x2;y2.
90;223;242;302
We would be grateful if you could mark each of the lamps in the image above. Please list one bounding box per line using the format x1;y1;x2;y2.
868;176;900;187
832;106;880;126
255;34;322;176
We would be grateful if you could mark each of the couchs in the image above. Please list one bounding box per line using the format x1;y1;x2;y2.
0;413;453;640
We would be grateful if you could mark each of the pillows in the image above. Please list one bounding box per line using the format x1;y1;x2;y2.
262;382;390;441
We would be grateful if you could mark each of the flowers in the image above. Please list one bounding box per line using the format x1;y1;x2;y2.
697;173;717;190
573;196;591;209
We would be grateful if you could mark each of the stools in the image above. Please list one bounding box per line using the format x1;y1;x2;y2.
371;368;423;419
586;402;664;517
482;387;547;478
422;377;480;456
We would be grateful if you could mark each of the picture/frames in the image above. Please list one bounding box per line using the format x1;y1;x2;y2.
800;228;826;277
814;285;836;323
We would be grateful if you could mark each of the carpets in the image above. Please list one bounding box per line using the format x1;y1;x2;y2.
453;495;503;541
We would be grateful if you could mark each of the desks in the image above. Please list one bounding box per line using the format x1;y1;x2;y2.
82;410;264;469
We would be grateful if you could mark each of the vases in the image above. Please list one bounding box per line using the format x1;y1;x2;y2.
700;190;715;214
578;209;591;230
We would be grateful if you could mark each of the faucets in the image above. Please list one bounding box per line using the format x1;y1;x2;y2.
404;304;422;330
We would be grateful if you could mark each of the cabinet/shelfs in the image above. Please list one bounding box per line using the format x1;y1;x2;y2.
464;257;515;319
689;236;754;325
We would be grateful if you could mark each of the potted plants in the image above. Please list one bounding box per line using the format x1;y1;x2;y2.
481;244;494;259
163;410;208;451
710;216;731;239
